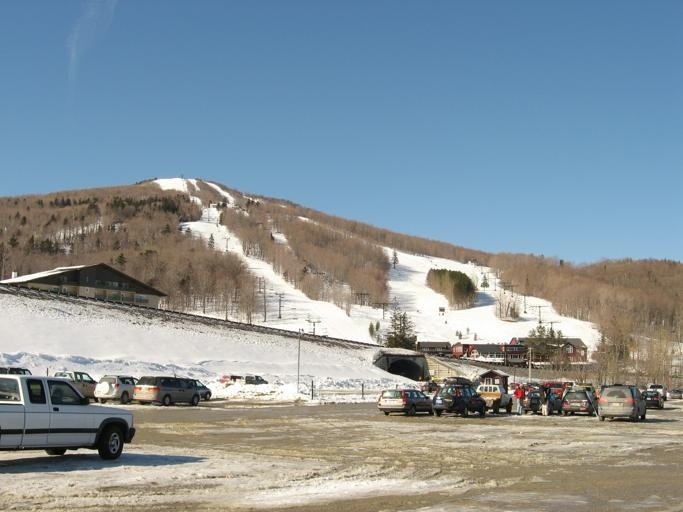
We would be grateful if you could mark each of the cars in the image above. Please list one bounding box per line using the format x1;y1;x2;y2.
219;374;241;384
376;388;432;417
515;381;667;415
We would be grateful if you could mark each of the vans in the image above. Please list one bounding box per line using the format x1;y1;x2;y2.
0;364;212;407
596;384;648;421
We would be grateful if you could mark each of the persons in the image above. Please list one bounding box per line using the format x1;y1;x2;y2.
540;388;551;416
513;384;526;415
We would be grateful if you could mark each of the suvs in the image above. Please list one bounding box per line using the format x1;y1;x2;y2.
429;377;487;417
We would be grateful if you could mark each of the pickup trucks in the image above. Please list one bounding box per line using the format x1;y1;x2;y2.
0;373;136;463
235;373;268;386
473;383;514;414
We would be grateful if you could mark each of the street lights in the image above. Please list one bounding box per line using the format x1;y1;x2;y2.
296;327;304;391
